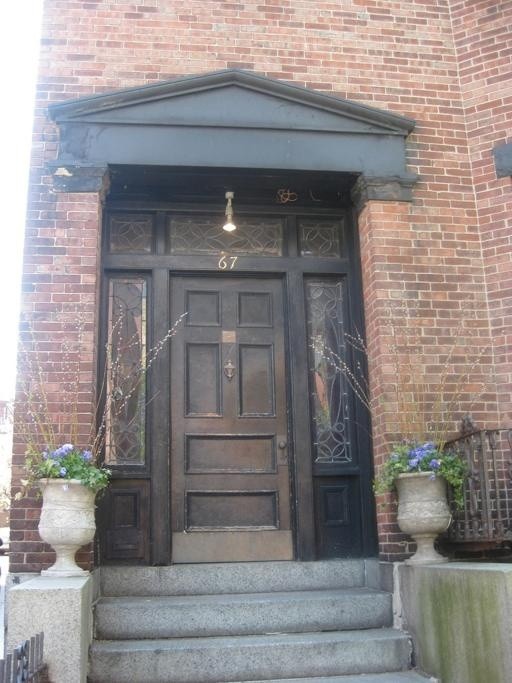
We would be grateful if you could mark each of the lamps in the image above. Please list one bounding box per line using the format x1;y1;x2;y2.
221;192;237;231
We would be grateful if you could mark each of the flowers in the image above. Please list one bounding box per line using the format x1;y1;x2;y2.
309;290;498;508
7;296;189;510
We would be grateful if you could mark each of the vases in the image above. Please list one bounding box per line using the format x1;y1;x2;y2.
36;476;97;576
394;470;453;565
